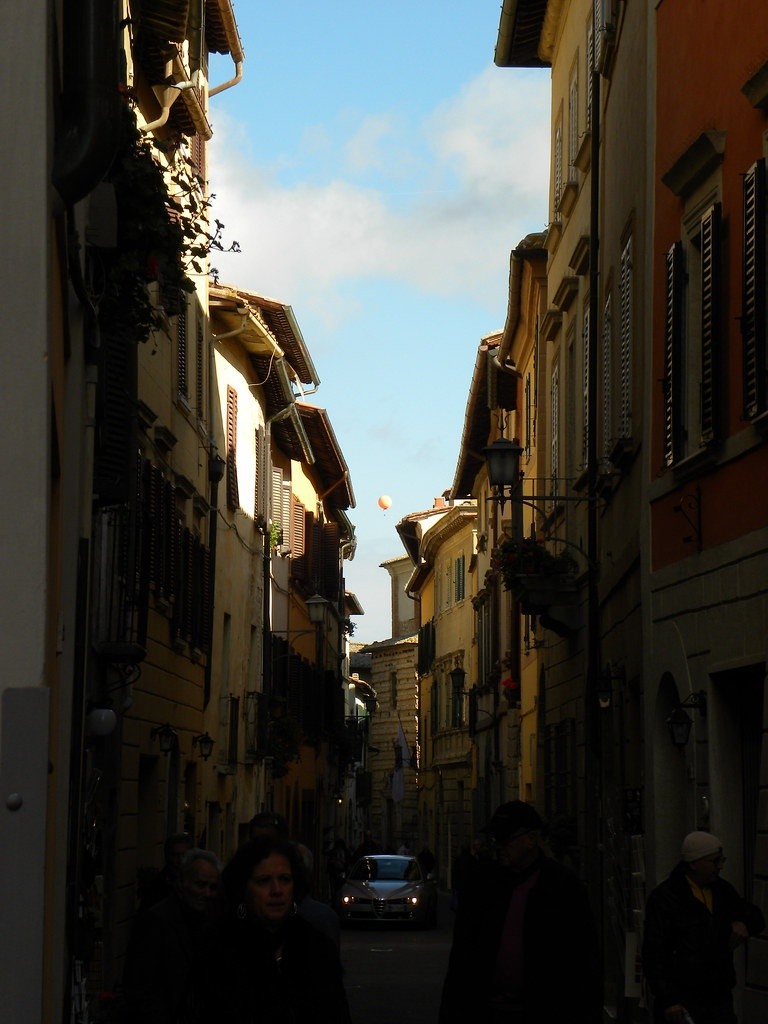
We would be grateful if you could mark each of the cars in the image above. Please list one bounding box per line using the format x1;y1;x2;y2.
339;854;442;926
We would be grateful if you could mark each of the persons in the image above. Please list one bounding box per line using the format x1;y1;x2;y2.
119;799;766;1024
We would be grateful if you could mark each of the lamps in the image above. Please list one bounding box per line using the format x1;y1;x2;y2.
304;583;330;626
207;449;227;486
191;731;217;763
665;688;707;756
449;658;468;691
149;722;180;759
482;405;526;518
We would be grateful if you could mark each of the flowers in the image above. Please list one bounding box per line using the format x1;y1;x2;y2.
147;254;158;285
489;534;545;591
502;677;519;709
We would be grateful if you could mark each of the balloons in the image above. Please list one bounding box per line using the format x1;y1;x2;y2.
377;495;392;509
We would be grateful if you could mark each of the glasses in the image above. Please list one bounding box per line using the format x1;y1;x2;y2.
699;855;727;863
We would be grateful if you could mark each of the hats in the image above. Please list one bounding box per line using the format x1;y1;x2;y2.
681;832;724;861
479;800;543;843
